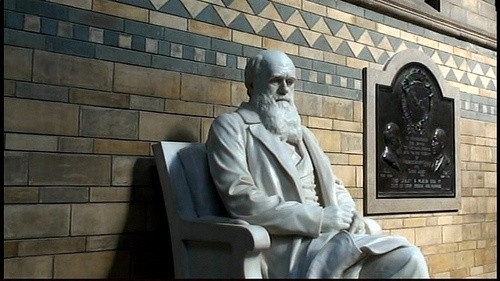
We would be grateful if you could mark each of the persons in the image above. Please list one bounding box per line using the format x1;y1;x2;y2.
429;128;452;179
381;121;402;172
204;49;430;280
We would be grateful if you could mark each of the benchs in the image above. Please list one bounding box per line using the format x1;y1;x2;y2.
150;139;386;280
